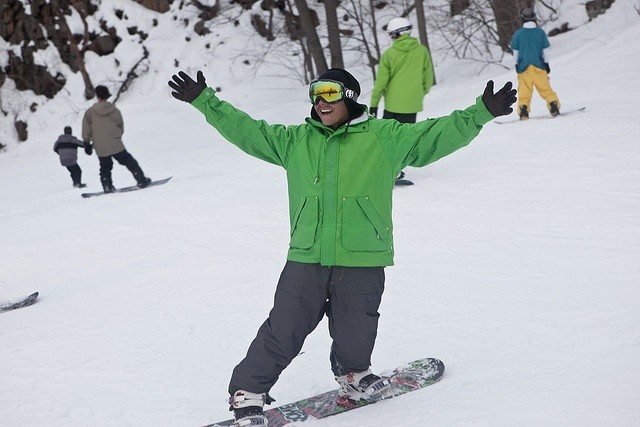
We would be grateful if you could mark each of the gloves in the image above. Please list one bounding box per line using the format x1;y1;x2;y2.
169;71;206;103
546;63;550;73
482;82;516;117
369;106;378;115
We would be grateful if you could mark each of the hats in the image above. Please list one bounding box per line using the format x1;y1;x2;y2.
318;68;361;113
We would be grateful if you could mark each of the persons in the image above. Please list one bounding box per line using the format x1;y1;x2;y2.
53;127;93;188
167;68;517;426
368;17;434;182
82;85;151;193
510;8;561;120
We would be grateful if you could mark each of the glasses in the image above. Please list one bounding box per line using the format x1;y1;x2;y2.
308;80;346;104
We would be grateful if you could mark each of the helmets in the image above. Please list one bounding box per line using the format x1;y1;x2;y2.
521;8;535;22
387;17;414;40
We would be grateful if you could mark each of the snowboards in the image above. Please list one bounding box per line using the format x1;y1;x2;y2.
200;357;444;427
0;291;39;313
494;107;586;124
81;176;173;197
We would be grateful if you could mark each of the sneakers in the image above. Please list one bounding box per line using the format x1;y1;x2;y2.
104;185;118;194
548;102;558;116
138;176;151;188
229;390;267;427
334;369;388;398
518;106;529;120
